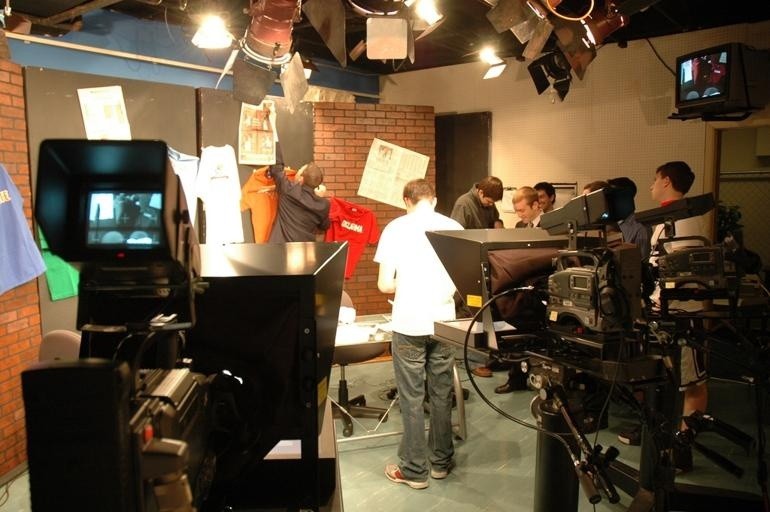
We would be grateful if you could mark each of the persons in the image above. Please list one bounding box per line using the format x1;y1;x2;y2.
581;182;625;243
120;196;140;225
264;101;330;243
495;186;543;394
687;51;726;100
373;178;465;489
378;145;392;168
534;182;556;213
450;175;506;377
608;176;649;263
648;160;709;431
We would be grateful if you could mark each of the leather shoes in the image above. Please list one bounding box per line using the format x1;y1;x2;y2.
495;383;511;393
471;367;492;377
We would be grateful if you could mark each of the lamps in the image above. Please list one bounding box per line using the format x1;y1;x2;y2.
184;1;657;105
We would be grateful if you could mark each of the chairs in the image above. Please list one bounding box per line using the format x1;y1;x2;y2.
332;342;392;435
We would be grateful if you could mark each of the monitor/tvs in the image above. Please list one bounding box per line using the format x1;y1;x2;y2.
675;42;770;114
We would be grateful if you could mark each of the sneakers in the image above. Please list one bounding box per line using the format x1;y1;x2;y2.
385;464;447;489
